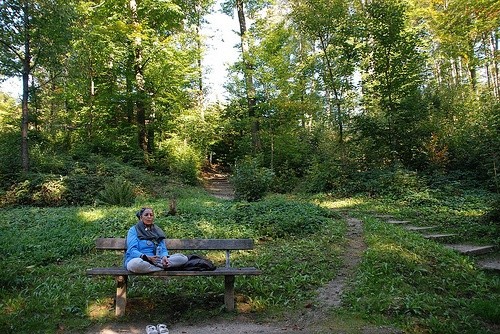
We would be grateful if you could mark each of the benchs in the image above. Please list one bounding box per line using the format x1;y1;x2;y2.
84;239;262;316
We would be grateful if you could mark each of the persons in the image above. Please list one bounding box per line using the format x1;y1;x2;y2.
123;208;188;273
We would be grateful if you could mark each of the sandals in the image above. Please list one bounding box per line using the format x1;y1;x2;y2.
146;325;158;334
157;324;169;334
